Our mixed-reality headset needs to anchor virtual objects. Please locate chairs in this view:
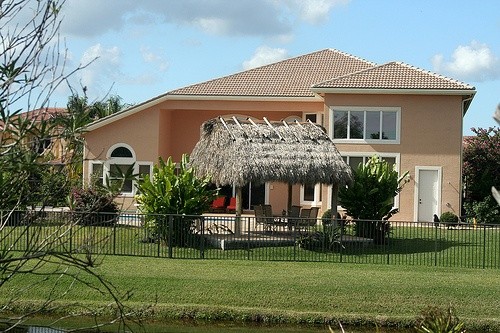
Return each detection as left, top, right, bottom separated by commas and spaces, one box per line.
227, 198, 236, 213
254, 206, 266, 233
211, 196, 226, 213
285, 206, 301, 231
294, 209, 311, 232
262, 205, 284, 235
301, 207, 320, 233
